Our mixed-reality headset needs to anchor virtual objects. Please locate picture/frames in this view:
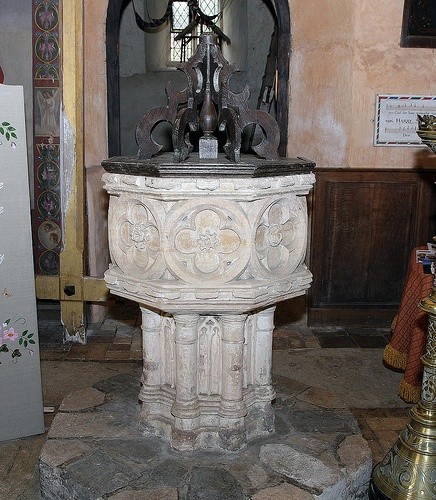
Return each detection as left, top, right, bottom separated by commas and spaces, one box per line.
399, 0, 436, 49
